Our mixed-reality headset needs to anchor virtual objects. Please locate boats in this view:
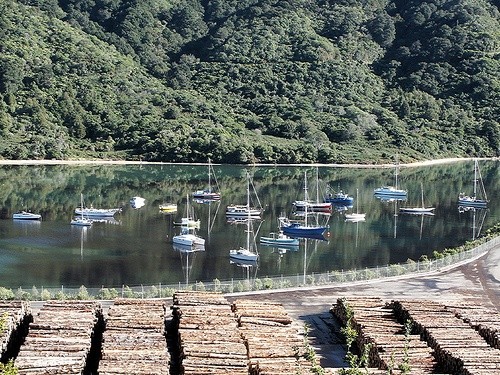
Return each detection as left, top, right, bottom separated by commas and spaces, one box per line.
344, 212, 366, 219
159, 203, 177, 209
159, 209, 177, 215
172, 216, 200, 229
92, 217, 123, 224
69, 218, 93, 226
292, 211, 332, 219
374, 186, 408, 196
191, 189, 221, 200
13, 218, 41, 225
331, 202, 353, 214
131, 204, 145, 209
374, 192, 408, 204
12, 210, 43, 222
226, 203, 262, 215
173, 234, 205, 245
278, 217, 327, 235
192, 197, 221, 204
260, 230, 299, 246
130, 196, 146, 204
225, 215, 261, 225
229, 247, 259, 260
323, 193, 353, 203
229, 257, 258, 269
457, 203, 489, 213
292, 200, 332, 212
456, 193, 492, 209
75, 207, 122, 216
344, 218, 366, 223
172, 242, 206, 252
399, 205, 436, 212
